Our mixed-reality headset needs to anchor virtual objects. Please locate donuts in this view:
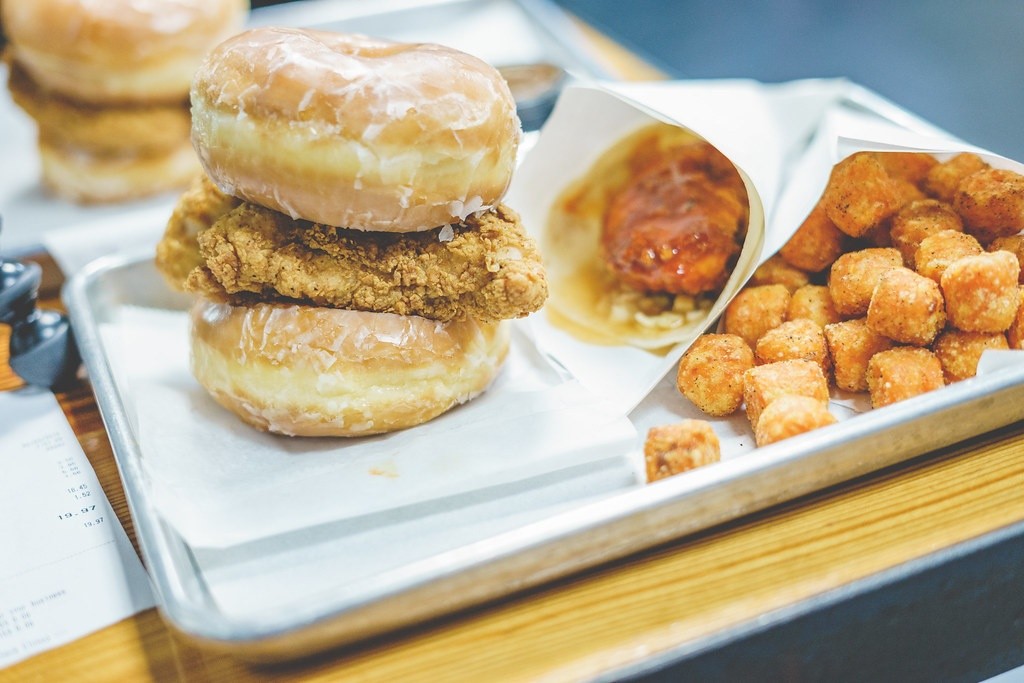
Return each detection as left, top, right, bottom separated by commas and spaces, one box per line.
0, 0, 551, 436
